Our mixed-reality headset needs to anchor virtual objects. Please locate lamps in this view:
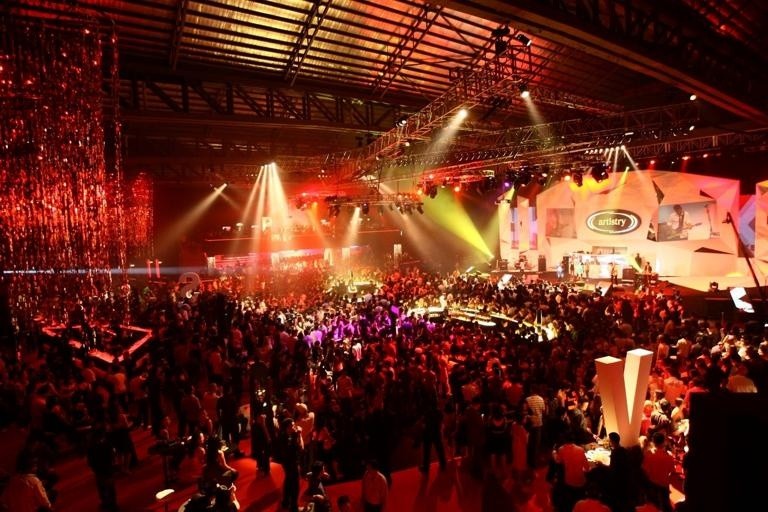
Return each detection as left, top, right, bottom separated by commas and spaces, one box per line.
516, 33, 532, 47
518, 84, 529, 97
